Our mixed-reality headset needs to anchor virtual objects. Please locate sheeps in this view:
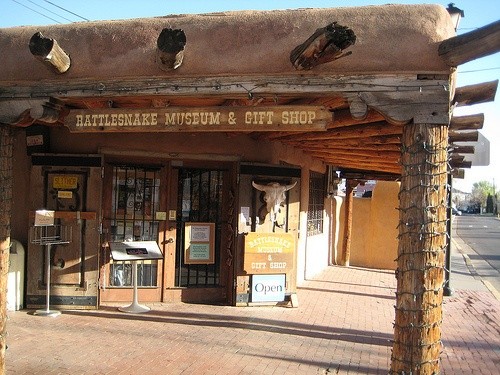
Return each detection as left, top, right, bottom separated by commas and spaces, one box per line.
252, 180, 298, 223
